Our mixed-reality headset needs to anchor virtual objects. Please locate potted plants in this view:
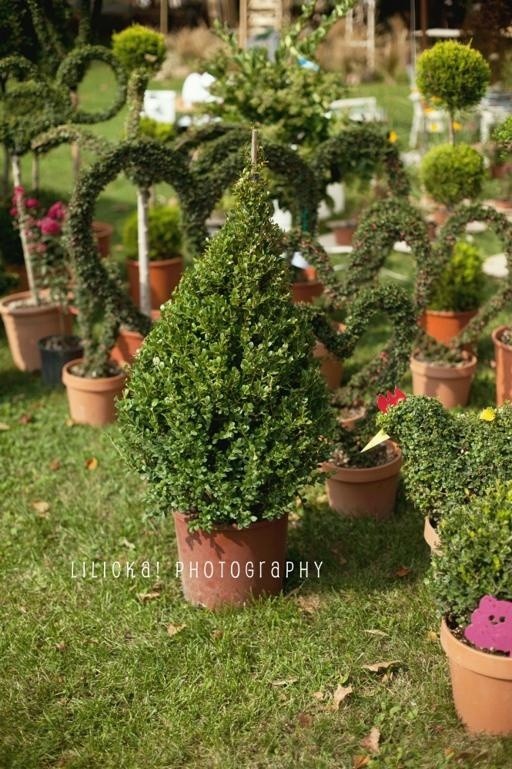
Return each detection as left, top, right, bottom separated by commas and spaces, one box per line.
0, 0, 512, 743
